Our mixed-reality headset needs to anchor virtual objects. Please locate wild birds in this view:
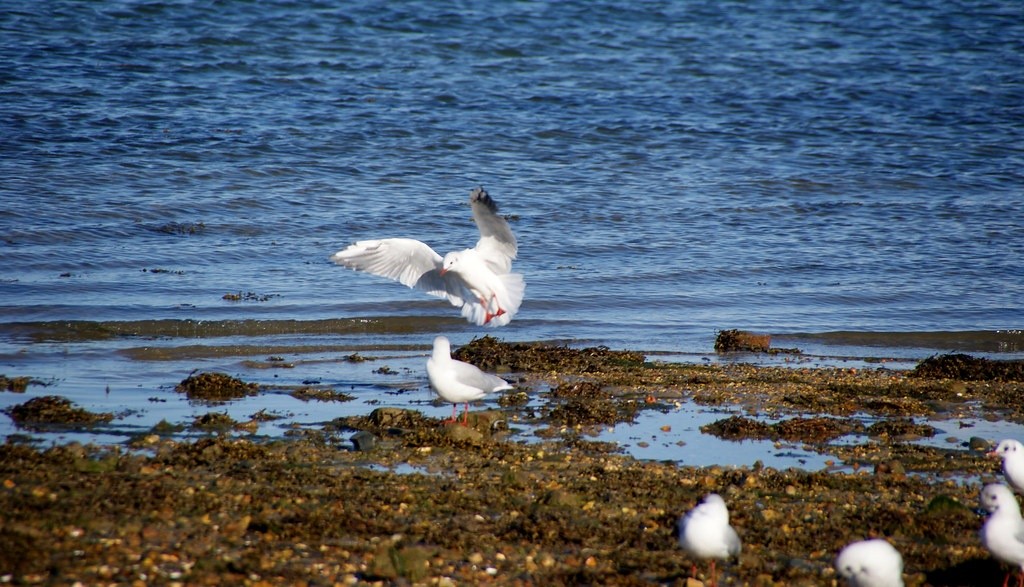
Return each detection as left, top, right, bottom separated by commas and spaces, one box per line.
676, 492, 743, 587
977, 484, 1024, 587
424, 334, 514, 427
833, 538, 905, 587
325, 186, 527, 327
985, 438, 1024, 493
